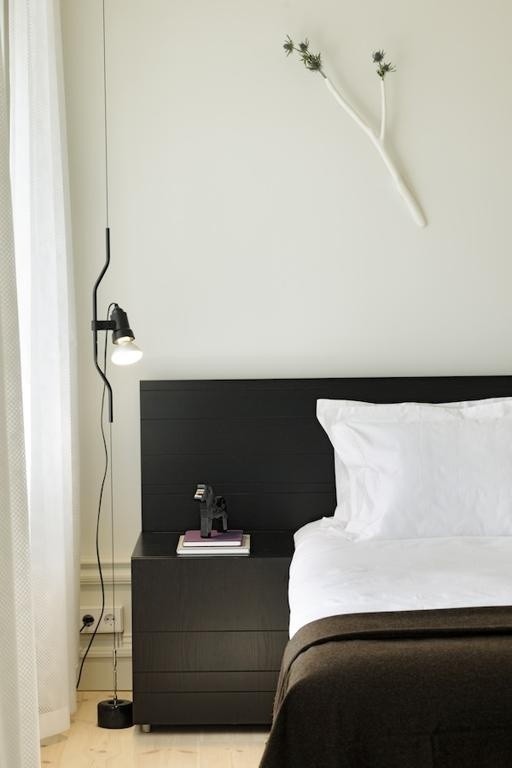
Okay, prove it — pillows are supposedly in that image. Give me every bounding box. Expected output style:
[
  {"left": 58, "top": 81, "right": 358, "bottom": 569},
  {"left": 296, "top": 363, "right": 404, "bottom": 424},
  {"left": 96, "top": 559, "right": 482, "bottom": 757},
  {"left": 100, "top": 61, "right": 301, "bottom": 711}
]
[{"left": 311, "top": 395, "right": 511, "bottom": 539}]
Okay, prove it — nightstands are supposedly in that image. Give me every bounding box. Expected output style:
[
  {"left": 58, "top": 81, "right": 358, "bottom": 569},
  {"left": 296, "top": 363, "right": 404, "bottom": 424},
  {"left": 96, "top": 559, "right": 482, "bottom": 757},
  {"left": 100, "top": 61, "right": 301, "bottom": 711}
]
[{"left": 130, "top": 530, "right": 294, "bottom": 733}]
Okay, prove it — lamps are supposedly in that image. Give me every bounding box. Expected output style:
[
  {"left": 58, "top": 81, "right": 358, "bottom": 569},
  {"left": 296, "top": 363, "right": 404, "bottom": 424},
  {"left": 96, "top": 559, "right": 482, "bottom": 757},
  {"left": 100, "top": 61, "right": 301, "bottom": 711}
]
[{"left": 87, "top": 228, "right": 141, "bottom": 420}]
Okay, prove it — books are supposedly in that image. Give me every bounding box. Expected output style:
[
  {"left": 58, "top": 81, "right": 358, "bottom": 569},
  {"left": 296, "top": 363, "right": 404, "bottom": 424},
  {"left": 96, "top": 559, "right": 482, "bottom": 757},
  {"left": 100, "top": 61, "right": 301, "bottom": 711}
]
[
  {"left": 182, "top": 528, "right": 243, "bottom": 547},
  {"left": 175, "top": 533, "right": 251, "bottom": 555}
]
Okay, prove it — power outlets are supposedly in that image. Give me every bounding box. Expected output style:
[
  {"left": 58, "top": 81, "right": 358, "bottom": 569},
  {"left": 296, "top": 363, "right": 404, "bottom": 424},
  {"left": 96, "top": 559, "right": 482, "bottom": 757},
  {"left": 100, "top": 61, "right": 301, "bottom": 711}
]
[{"left": 79, "top": 607, "right": 124, "bottom": 634}]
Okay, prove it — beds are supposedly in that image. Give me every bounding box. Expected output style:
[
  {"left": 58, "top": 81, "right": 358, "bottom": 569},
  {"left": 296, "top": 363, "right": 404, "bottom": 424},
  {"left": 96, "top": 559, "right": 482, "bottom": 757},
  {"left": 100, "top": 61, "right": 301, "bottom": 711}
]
[{"left": 139, "top": 378, "right": 511, "bottom": 768}]
